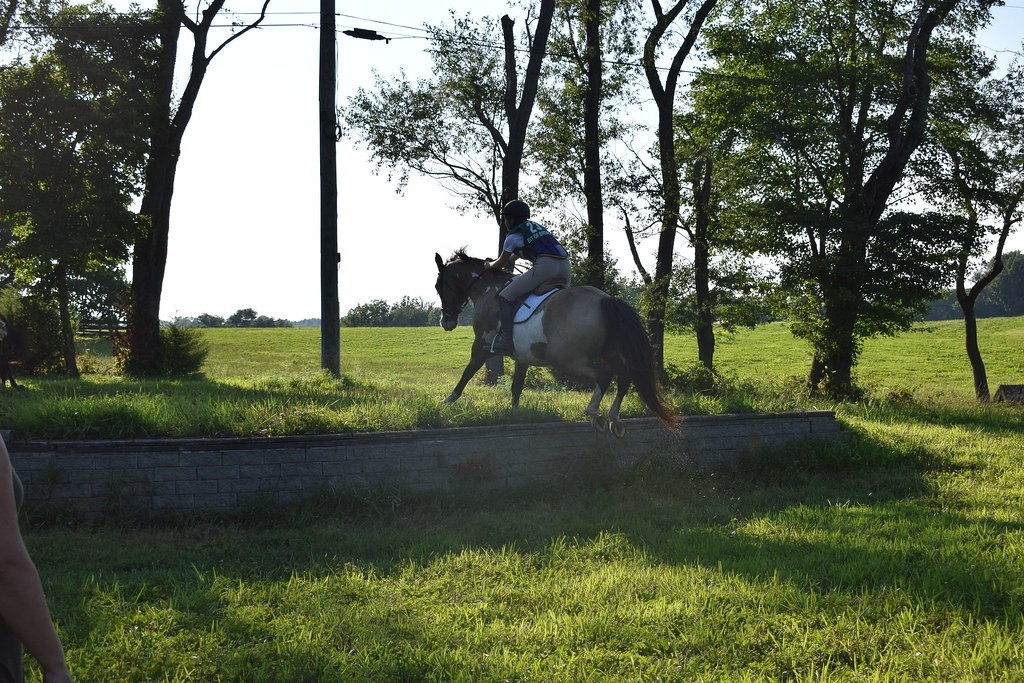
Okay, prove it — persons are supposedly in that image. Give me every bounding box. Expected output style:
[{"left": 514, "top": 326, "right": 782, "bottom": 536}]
[
  {"left": 482, "top": 200, "right": 570, "bottom": 357},
  {"left": 0, "top": 431, "right": 73, "bottom": 683}
]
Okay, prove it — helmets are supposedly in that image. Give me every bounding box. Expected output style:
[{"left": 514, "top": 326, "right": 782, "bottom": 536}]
[{"left": 500, "top": 200, "right": 531, "bottom": 219}]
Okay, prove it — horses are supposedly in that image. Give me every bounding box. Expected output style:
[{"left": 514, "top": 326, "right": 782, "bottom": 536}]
[{"left": 434, "top": 248, "right": 688, "bottom": 441}]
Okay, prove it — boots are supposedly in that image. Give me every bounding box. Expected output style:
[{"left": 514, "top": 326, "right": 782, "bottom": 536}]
[{"left": 482, "top": 295, "right": 517, "bottom": 351}]
